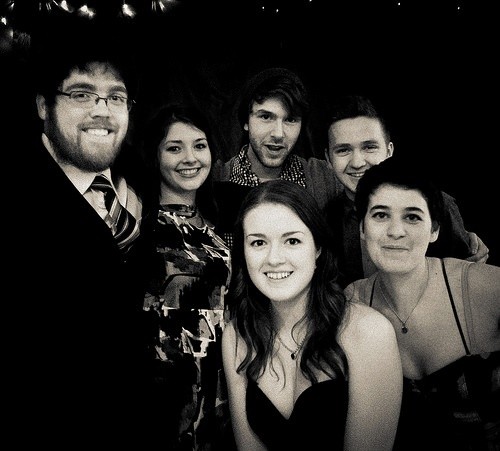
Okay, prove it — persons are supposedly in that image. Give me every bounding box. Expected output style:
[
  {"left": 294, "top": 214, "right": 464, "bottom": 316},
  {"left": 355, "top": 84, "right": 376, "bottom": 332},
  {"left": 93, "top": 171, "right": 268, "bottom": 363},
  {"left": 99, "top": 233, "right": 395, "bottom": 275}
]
[
  {"left": 221, "top": 180, "right": 404, "bottom": 451},
  {"left": 212, "top": 68, "right": 490, "bottom": 263},
  {"left": 342, "top": 157, "right": 500, "bottom": 451},
  {"left": 319, "top": 94, "right": 472, "bottom": 289},
  {"left": 0, "top": 47, "right": 236, "bottom": 451}
]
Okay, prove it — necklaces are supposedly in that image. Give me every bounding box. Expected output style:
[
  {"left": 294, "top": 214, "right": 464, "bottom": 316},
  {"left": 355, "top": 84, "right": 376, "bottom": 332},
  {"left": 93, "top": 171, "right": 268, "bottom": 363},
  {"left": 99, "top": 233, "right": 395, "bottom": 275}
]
[
  {"left": 375, "top": 257, "right": 431, "bottom": 333},
  {"left": 273, "top": 324, "right": 309, "bottom": 360}
]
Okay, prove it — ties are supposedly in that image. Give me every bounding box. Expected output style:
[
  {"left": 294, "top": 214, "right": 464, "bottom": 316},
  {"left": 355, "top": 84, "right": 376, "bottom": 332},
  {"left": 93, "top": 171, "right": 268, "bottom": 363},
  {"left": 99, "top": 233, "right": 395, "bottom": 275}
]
[{"left": 89, "top": 176, "right": 140, "bottom": 254}]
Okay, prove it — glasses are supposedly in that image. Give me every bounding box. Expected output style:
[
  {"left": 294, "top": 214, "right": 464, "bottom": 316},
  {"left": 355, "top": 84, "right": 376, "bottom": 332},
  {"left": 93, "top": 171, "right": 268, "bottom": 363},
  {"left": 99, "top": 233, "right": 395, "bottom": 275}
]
[{"left": 56, "top": 90, "right": 136, "bottom": 113}]
